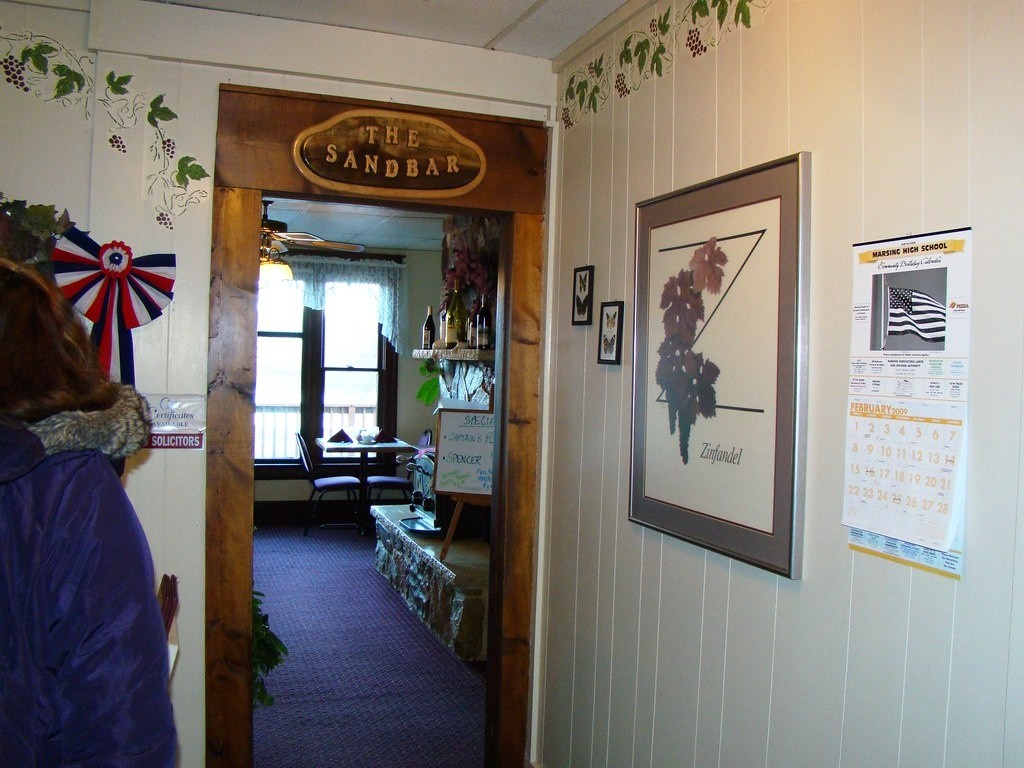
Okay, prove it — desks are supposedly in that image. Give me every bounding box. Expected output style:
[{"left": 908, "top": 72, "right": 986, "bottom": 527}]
[{"left": 314, "top": 437, "right": 416, "bottom": 528}]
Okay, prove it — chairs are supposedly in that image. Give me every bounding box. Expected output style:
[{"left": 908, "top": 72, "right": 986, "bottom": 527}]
[
  {"left": 294, "top": 432, "right": 368, "bottom": 538},
  {"left": 360, "top": 428, "right": 432, "bottom": 531}
]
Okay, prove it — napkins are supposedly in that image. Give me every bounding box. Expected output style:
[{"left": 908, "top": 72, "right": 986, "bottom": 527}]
[{"left": 328, "top": 429, "right": 352, "bottom": 442}]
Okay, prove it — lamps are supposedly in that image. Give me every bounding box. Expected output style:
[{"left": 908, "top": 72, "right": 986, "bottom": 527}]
[{"left": 259, "top": 227, "right": 295, "bottom": 284}]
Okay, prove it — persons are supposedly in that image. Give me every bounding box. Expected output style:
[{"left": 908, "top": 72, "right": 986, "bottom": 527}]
[{"left": 0, "top": 256, "right": 178, "bottom": 768}]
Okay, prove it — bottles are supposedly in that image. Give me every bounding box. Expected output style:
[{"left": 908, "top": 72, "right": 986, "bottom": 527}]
[
  {"left": 465, "top": 292, "right": 492, "bottom": 349},
  {"left": 439, "top": 279, "right": 462, "bottom": 349},
  {"left": 422, "top": 306, "right": 436, "bottom": 350}
]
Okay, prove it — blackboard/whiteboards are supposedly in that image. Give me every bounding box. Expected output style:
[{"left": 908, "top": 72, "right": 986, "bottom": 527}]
[{"left": 432, "top": 409, "right": 495, "bottom": 506}]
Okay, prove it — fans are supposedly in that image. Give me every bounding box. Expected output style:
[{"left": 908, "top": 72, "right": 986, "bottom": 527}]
[{"left": 256, "top": 199, "right": 368, "bottom": 255}]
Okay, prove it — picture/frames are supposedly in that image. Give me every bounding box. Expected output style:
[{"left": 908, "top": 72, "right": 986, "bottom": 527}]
[
  {"left": 628, "top": 152, "right": 812, "bottom": 580},
  {"left": 572, "top": 265, "right": 595, "bottom": 325},
  {"left": 595, "top": 300, "right": 625, "bottom": 365}
]
